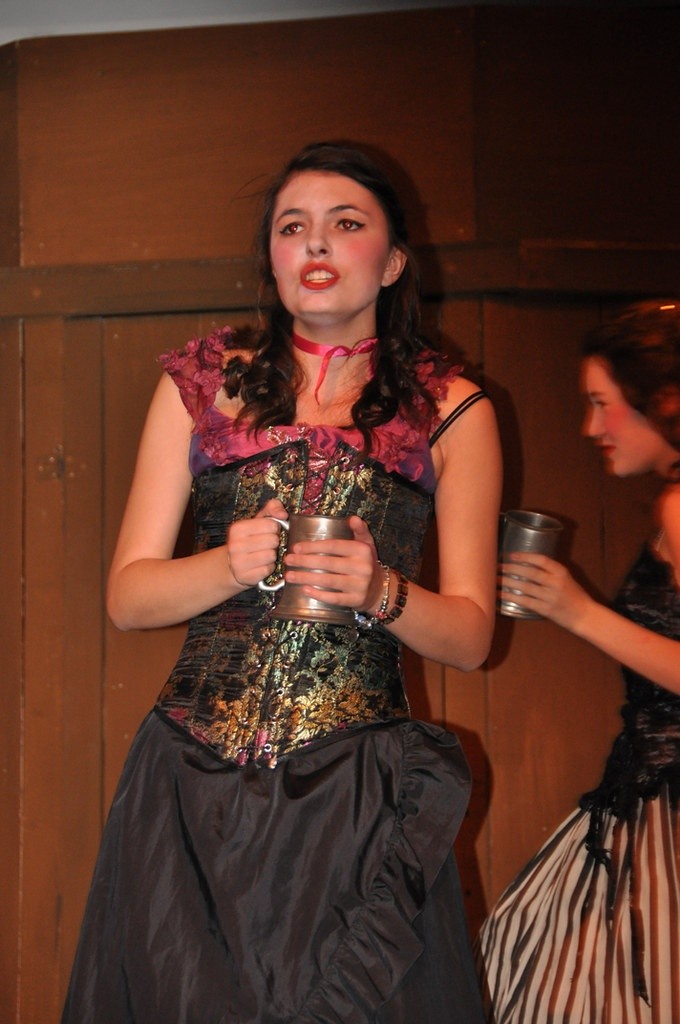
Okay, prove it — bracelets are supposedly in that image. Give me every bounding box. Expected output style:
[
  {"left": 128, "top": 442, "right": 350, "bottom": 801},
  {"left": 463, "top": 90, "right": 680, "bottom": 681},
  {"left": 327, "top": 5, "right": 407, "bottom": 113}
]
[
  {"left": 226, "top": 544, "right": 256, "bottom": 588},
  {"left": 353, "top": 561, "right": 408, "bottom": 629}
]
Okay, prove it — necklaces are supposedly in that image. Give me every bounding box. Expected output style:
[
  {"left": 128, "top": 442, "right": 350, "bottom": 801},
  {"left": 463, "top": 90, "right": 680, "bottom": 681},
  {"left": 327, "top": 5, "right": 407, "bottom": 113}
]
[{"left": 289, "top": 325, "right": 377, "bottom": 408}]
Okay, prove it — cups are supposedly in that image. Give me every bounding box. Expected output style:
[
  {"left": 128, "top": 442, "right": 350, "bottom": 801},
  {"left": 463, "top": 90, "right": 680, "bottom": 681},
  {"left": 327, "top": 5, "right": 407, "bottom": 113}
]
[
  {"left": 497, "top": 508, "right": 563, "bottom": 621},
  {"left": 256, "top": 513, "right": 359, "bottom": 626}
]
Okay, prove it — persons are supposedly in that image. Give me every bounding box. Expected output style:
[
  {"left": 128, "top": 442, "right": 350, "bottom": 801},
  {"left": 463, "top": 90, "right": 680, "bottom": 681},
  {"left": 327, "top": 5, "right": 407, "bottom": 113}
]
[
  {"left": 61, "top": 143, "right": 504, "bottom": 1023},
  {"left": 472, "top": 298, "right": 680, "bottom": 1023}
]
